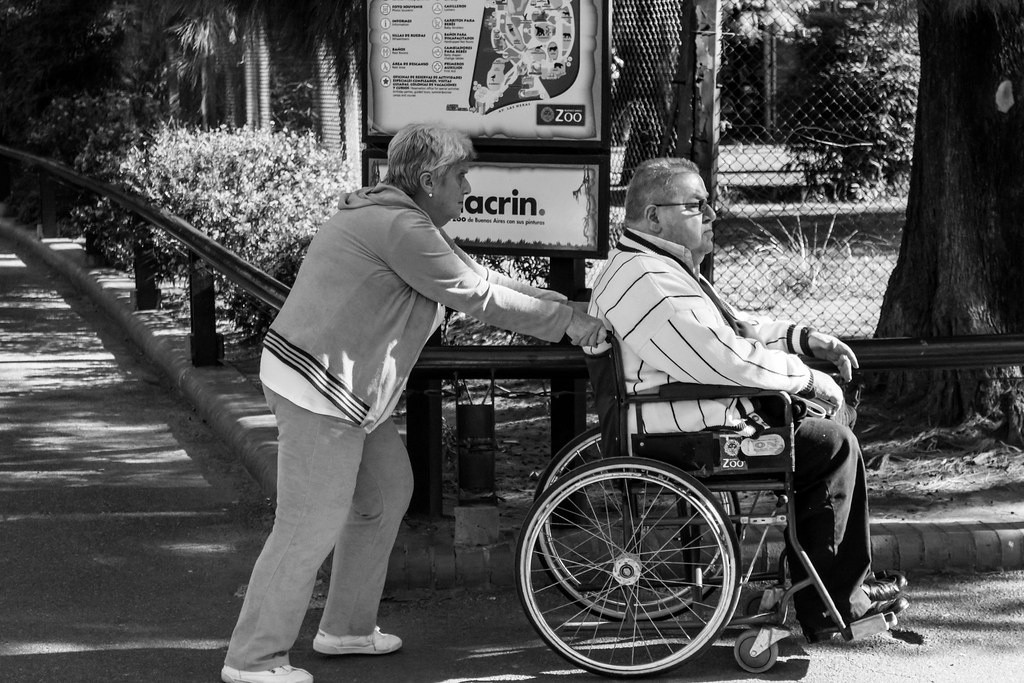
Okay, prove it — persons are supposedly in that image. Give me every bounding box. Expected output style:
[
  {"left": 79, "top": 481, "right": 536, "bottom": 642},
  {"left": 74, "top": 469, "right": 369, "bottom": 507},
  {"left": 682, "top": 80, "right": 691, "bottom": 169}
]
[
  {"left": 219, "top": 122, "right": 609, "bottom": 682},
  {"left": 581, "top": 156, "right": 911, "bottom": 647}
]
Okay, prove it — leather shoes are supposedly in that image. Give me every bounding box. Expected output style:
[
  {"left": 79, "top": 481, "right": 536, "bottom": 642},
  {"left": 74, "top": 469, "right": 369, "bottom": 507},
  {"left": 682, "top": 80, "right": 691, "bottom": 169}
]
[
  {"left": 806, "top": 597, "right": 909, "bottom": 644},
  {"left": 861, "top": 573, "right": 907, "bottom": 602}
]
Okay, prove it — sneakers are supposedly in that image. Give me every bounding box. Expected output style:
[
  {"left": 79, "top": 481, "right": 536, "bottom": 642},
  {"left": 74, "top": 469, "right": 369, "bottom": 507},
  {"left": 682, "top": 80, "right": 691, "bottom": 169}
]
[
  {"left": 313, "top": 625, "right": 403, "bottom": 654},
  {"left": 221, "top": 663, "right": 313, "bottom": 683}
]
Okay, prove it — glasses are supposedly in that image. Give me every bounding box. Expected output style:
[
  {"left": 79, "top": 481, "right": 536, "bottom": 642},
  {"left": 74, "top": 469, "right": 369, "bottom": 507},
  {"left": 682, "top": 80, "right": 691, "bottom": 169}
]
[{"left": 651, "top": 199, "right": 713, "bottom": 213}]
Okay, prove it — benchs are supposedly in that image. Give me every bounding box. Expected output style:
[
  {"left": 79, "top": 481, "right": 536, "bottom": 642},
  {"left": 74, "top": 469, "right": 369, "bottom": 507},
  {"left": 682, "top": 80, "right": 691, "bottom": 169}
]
[{"left": 608, "top": 143, "right": 907, "bottom": 225}]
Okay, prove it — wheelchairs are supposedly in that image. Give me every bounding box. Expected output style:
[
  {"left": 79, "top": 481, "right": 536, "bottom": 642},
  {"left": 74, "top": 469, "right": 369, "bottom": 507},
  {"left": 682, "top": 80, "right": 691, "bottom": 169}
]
[{"left": 510, "top": 332, "right": 923, "bottom": 678}]
